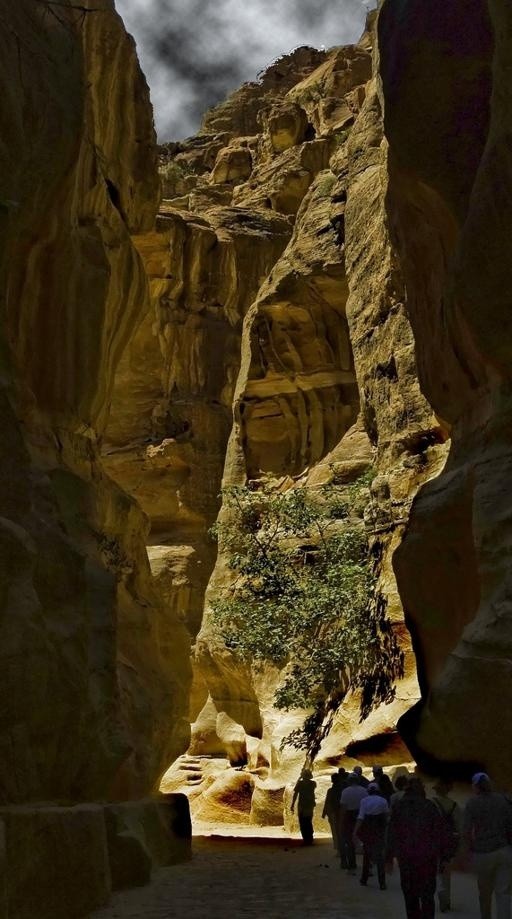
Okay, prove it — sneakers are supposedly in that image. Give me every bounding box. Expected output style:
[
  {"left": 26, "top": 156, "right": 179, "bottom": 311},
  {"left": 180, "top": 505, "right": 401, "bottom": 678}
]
[{"left": 343, "top": 868, "right": 394, "bottom": 892}]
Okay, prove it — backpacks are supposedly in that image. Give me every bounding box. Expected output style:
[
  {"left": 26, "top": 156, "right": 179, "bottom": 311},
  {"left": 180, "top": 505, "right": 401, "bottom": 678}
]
[{"left": 432, "top": 797, "right": 460, "bottom": 864}]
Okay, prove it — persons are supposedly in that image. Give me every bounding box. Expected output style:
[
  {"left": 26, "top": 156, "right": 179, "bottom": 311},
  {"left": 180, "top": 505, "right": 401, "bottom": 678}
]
[
  {"left": 462, "top": 772, "right": 511, "bottom": 918},
  {"left": 384, "top": 777, "right": 442, "bottom": 918},
  {"left": 321, "top": 765, "right": 406, "bottom": 891},
  {"left": 427, "top": 779, "right": 464, "bottom": 913},
  {"left": 289, "top": 769, "right": 317, "bottom": 845}
]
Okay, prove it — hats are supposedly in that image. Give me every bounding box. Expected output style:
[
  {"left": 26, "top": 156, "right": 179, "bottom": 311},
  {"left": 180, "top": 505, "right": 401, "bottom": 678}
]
[
  {"left": 471, "top": 772, "right": 490, "bottom": 786},
  {"left": 366, "top": 782, "right": 380, "bottom": 793},
  {"left": 432, "top": 777, "right": 453, "bottom": 790},
  {"left": 373, "top": 764, "right": 383, "bottom": 773},
  {"left": 353, "top": 766, "right": 363, "bottom": 775},
  {"left": 301, "top": 769, "right": 313, "bottom": 779},
  {"left": 344, "top": 773, "right": 361, "bottom": 786},
  {"left": 404, "top": 778, "right": 425, "bottom": 791}
]
